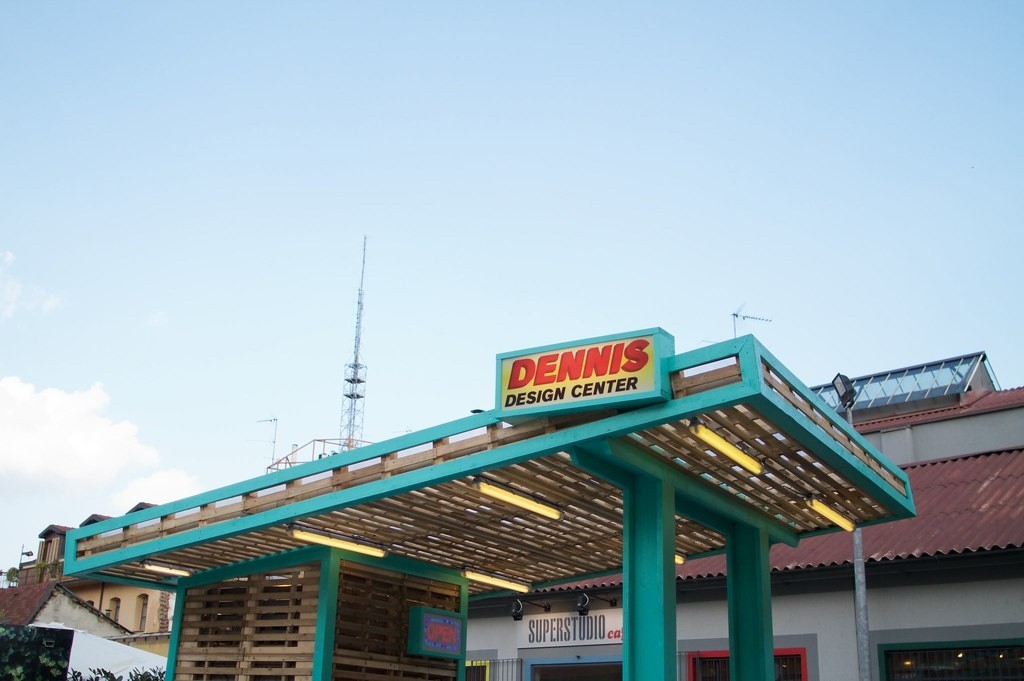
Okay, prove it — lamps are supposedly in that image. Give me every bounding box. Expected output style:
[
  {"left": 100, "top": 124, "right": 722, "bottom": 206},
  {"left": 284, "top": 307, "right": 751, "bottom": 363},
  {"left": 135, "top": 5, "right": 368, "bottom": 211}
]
[
  {"left": 510, "top": 598, "right": 551, "bottom": 621},
  {"left": 577, "top": 593, "right": 616, "bottom": 616}
]
